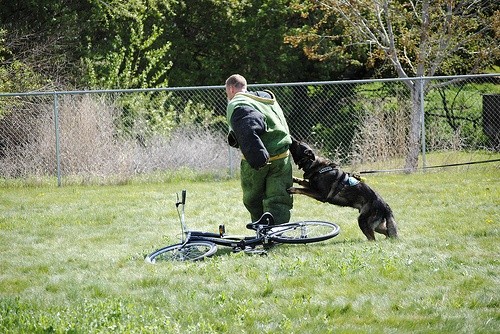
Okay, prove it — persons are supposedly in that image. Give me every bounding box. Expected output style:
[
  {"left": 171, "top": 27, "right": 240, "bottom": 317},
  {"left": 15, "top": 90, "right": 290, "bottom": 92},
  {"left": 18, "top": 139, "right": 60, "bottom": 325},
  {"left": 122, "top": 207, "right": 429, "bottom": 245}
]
[{"left": 224, "top": 73, "right": 294, "bottom": 225}]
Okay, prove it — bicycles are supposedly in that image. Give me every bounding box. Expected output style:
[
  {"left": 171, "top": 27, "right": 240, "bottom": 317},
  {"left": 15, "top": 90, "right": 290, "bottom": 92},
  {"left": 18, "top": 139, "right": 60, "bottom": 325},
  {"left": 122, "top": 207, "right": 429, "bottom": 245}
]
[{"left": 144, "top": 189, "right": 340, "bottom": 267}]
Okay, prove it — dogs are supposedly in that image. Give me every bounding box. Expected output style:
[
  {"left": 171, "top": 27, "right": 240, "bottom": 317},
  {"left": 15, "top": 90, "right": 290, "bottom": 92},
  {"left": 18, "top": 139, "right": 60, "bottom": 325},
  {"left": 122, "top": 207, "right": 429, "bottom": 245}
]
[{"left": 287, "top": 137, "right": 397, "bottom": 241}]
